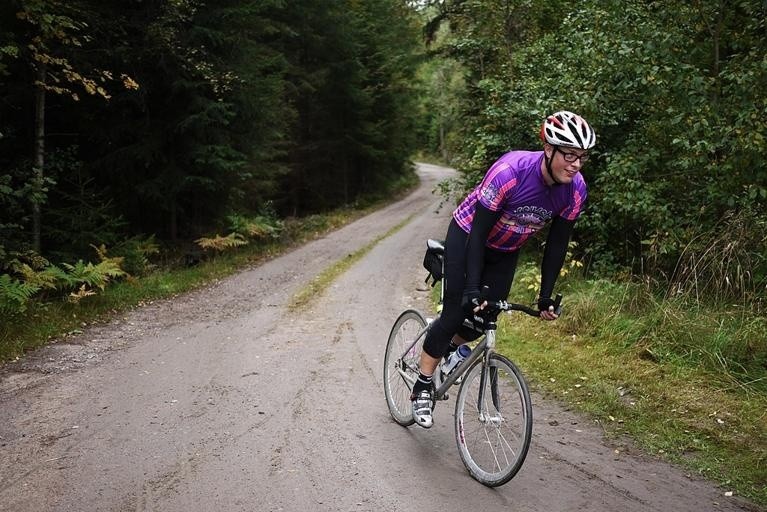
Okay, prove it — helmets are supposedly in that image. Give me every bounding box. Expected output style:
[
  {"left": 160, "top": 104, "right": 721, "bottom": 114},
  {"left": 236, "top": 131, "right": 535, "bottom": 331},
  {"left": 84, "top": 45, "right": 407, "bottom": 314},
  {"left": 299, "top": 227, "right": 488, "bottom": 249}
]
[{"left": 540, "top": 111, "right": 596, "bottom": 150}]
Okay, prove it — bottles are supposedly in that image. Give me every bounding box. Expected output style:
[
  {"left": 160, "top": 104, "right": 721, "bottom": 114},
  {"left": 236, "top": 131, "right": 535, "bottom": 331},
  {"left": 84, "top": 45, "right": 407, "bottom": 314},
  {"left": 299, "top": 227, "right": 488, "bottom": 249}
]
[{"left": 441, "top": 344, "right": 472, "bottom": 376}]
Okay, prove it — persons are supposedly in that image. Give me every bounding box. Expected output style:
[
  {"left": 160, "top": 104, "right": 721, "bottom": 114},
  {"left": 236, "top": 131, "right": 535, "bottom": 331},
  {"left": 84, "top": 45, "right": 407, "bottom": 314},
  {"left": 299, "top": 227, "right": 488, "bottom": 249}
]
[{"left": 411, "top": 111, "right": 599, "bottom": 429}]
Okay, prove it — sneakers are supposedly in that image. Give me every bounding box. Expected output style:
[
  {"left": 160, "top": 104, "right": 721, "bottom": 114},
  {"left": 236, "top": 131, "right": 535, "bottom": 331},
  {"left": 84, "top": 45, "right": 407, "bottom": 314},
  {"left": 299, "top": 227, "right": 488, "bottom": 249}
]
[
  {"left": 410, "top": 386, "right": 434, "bottom": 428},
  {"left": 442, "top": 354, "right": 463, "bottom": 384}
]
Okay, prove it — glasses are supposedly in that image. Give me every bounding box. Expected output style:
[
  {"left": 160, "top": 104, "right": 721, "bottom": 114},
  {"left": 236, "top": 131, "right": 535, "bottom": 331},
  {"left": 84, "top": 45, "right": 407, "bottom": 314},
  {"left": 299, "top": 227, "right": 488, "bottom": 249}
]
[{"left": 557, "top": 146, "right": 591, "bottom": 162}]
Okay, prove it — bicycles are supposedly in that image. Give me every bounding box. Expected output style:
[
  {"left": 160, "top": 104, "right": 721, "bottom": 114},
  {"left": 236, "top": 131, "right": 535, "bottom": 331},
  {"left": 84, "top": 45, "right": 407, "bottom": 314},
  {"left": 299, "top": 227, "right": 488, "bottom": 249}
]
[{"left": 382, "top": 239, "right": 562, "bottom": 487}]
